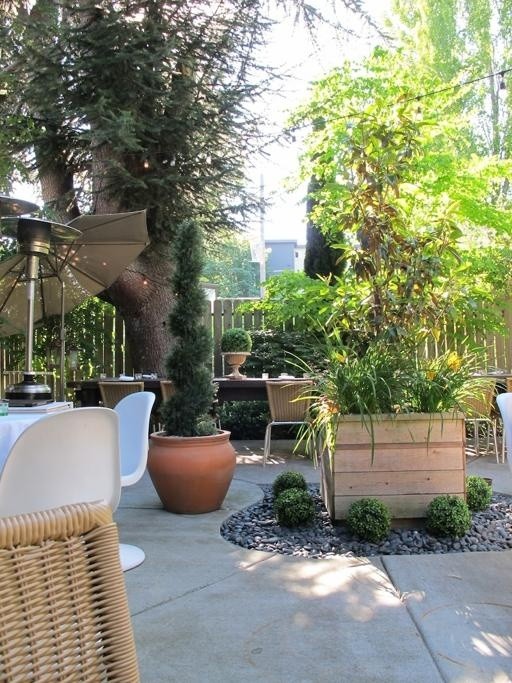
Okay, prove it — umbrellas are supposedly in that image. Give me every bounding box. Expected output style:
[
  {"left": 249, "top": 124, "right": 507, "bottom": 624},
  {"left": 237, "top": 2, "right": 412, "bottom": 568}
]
[{"left": 0, "top": 206, "right": 153, "bottom": 398}]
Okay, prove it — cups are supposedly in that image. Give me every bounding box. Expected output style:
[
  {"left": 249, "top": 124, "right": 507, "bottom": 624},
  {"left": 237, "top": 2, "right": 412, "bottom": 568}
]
[
  {"left": 261, "top": 371, "right": 311, "bottom": 378},
  {"left": 135, "top": 371, "right": 157, "bottom": 380},
  {"left": 100, "top": 372, "right": 106, "bottom": 379},
  {"left": 0, "top": 402, "right": 9, "bottom": 415}
]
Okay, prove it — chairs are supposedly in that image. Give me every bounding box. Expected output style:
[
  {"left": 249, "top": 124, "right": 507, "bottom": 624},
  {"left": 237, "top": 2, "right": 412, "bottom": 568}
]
[
  {"left": 108, "top": 390, "right": 159, "bottom": 571},
  {"left": 160, "top": 377, "right": 220, "bottom": 435},
  {"left": 263, "top": 374, "right": 314, "bottom": 461},
  {"left": 1, "top": 502, "right": 143, "bottom": 681},
  {"left": 1, "top": 407, "right": 124, "bottom": 525},
  {"left": 457, "top": 373, "right": 511, "bottom": 467},
  {"left": 103, "top": 380, "right": 143, "bottom": 405}
]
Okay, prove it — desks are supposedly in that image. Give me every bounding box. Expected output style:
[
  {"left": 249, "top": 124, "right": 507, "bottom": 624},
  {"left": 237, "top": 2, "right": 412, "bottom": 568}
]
[
  {"left": 1, "top": 403, "right": 74, "bottom": 468},
  {"left": 65, "top": 371, "right": 512, "bottom": 462}
]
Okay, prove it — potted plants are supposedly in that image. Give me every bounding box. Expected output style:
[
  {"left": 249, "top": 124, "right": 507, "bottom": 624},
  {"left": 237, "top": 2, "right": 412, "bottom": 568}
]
[
  {"left": 237, "top": 26, "right": 511, "bottom": 532},
  {"left": 147, "top": 222, "right": 237, "bottom": 517},
  {"left": 220, "top": 329, "right": 252, "bottom": 378}
]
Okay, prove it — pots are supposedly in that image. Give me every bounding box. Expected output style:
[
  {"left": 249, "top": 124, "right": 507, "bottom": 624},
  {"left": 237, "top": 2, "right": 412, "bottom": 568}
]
[{"left": 4, "top": 370, "right": 53, "bottom": 407}]
